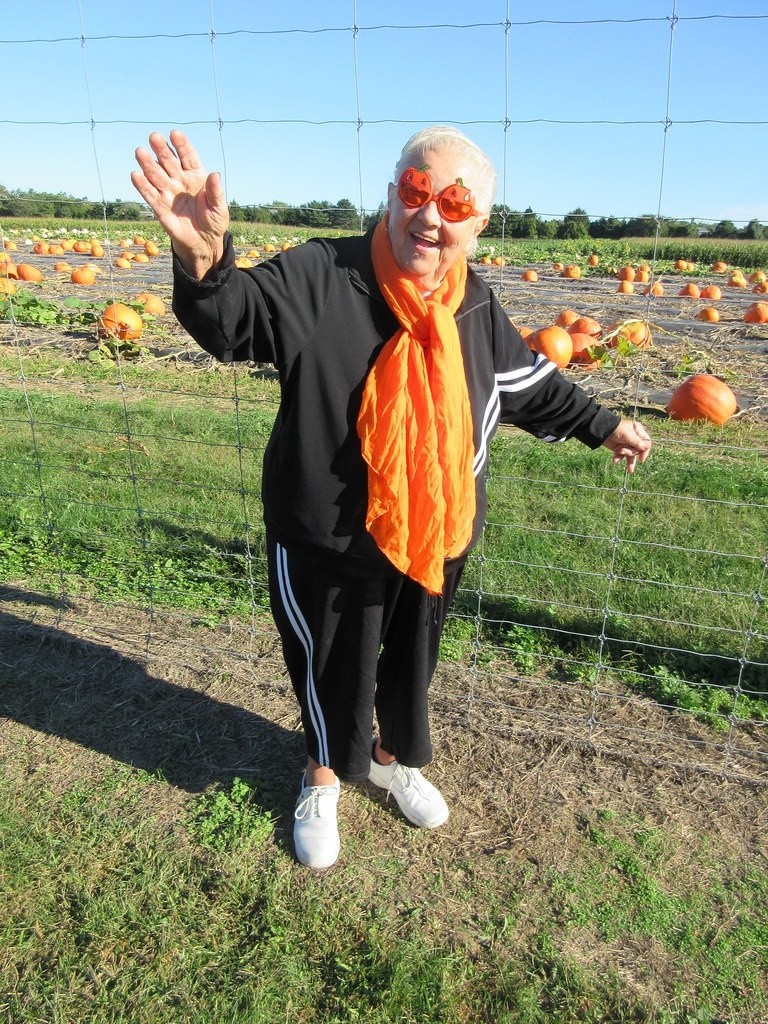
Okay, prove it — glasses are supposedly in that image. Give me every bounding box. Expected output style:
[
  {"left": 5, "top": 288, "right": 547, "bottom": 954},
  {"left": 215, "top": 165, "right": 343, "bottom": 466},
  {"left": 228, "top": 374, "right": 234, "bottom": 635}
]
[{"left": 397, "top": 165, "right": 484, "bottom": 222}]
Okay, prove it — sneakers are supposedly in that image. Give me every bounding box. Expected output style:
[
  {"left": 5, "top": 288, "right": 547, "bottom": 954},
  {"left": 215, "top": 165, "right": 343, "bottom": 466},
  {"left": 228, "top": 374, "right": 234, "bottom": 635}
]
[
  {"left": 293, "top": 769, "right": 340, "bottom": 868},
  {"left": 367, "top": 737, "right": 449, "bottom": 828}
]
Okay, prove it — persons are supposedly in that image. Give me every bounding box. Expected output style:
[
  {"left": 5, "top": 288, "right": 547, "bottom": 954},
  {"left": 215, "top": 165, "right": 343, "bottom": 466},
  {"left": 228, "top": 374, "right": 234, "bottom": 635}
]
[{"left": 129, "top": 125, "right": 652, "bottom": 872}]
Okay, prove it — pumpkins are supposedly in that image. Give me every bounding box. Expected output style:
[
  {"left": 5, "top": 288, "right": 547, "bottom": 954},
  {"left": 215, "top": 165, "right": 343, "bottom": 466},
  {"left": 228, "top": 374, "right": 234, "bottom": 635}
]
[
  {"left": 480, "top": 252, "right": 768, "bottom": 428},
  {"left": 0, "top": 227, "right": 166, "bottom": 341},
  {"left": 234, "top": 235, "right": 306, "bottom": 268}
]
